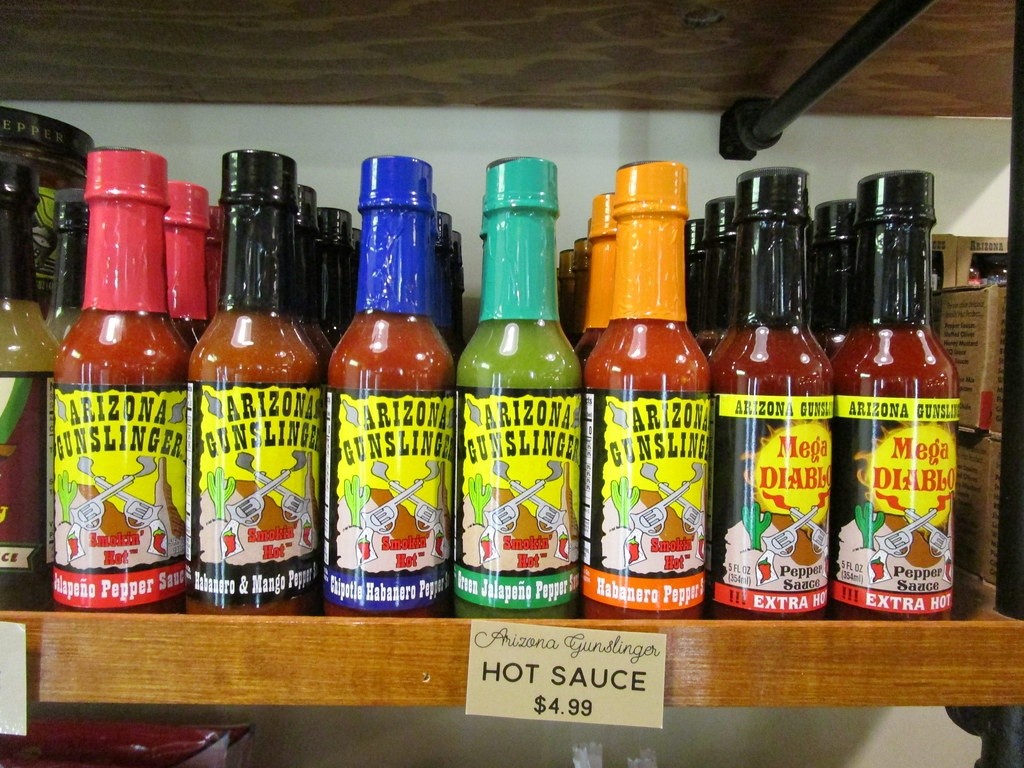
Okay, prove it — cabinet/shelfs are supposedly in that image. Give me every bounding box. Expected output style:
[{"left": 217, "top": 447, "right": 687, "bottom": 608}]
[{"left": 0, "top": 0, "right": 1024, "bottom": 711}]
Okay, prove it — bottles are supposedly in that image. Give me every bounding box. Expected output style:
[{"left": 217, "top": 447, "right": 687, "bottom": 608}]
[
  {"left": 969, "top": 269, "right": 1007, "bottom": 287},
  {"left": 0, "top": 149, "right": 959, "bottom": 620}
]
